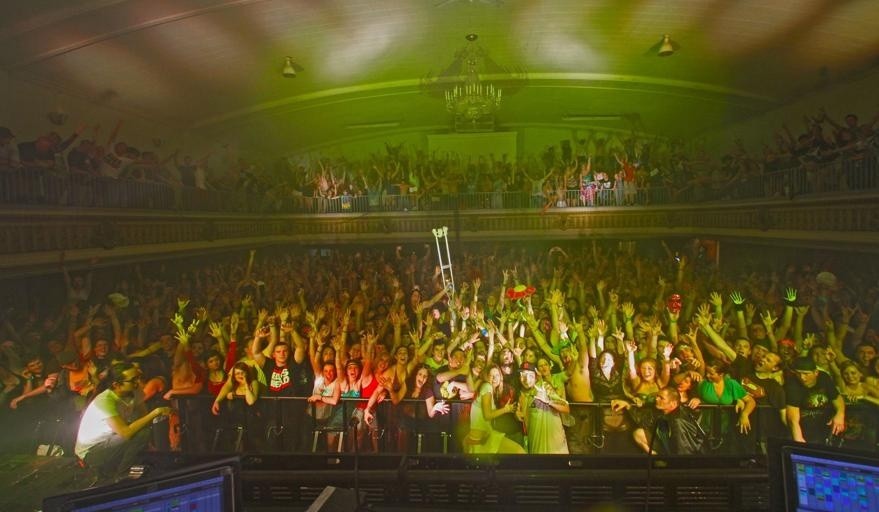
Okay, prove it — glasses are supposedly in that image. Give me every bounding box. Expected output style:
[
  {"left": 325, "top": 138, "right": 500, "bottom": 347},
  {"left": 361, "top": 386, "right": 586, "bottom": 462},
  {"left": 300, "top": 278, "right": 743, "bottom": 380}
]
[{"left": 118, "top": 377, "right": 139, "bottom": 383}]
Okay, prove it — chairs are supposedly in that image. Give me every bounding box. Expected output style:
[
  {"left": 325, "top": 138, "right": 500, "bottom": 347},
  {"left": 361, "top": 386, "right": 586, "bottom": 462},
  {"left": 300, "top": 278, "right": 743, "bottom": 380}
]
[{"left": 172, "top": 393, "right": 875, "bottom": 469}]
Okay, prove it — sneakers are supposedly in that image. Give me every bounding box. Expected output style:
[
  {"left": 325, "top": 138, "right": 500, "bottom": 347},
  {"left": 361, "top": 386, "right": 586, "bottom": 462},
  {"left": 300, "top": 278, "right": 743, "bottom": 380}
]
[{"left": 94, "top": 474, "right": 129, "bottom": 488}]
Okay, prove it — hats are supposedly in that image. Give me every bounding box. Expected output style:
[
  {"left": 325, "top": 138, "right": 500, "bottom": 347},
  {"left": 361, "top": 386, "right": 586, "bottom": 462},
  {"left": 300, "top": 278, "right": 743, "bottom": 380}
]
[
  {"left": 516, "top": 361, "right": 537, "bottom": 372},
  {"left": 754, "top": 338, "right": 771, "bottom": 351},
  {"left": 792, "top": 357, "right": 817, "bottom": 371}
]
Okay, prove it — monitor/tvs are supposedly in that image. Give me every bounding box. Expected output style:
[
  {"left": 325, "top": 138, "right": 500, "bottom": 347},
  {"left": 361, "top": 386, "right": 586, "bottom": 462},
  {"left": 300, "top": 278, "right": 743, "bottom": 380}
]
[
  {"left": 42, "top": 454, "right": 242, "bottom": 512},
  {"left": 773, "top": 439, "right": 879, "bottom": 512}
]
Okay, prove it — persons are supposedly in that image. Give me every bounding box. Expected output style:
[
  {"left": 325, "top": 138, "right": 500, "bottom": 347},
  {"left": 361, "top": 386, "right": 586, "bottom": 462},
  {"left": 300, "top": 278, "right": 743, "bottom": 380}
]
[{"left": 0, "top": 105, "right": 877, "bottom": 486}]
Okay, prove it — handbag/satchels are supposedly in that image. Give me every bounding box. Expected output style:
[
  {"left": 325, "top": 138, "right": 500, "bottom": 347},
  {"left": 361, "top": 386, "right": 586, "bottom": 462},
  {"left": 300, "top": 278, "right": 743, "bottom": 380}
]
[{"left": 464, "top": 430, "right": 490, "bottom": 445}]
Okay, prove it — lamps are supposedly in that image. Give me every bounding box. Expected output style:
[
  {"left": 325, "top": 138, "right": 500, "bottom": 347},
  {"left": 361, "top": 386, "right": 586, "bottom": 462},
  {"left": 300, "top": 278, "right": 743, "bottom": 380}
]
[
  {"left": 657, "top": 33, "right": 675, "bottom": 55},
  {"left": 417, "top": 34, "right": 529, "bottom": 122},
  {"left": 281, "top": 56, "right": 296, "bottom": 79}
]
